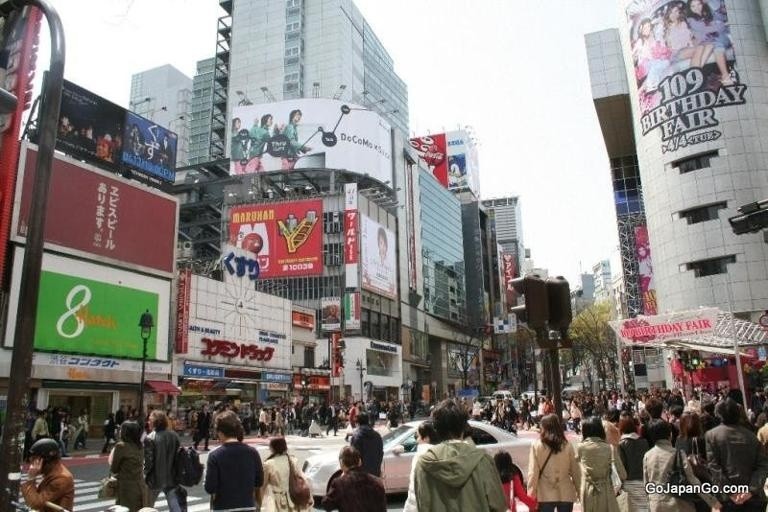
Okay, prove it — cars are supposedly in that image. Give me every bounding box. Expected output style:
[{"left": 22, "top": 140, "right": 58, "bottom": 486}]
[
  {"left": 298, "top": 417, "right": 534, "bottom": 500},
  {"left": 428, "top": 382, "right": 588, "bottom": 420}
]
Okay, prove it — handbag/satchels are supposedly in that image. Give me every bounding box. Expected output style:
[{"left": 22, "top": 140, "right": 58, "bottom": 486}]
[
  {"left": 661, "top": 465, "right": 697, "bottom": 500},
  {"left": 609, "top": 444, "right": 623, "bottom": 495},
  {"left": 287, "top": 451, "right": 311, "bottom": 505},
  {"left": 685, "top": 437, "right": 706, "bottom": 472},
  {"left": 98, "top": 474, "right": 119, "bottom": 500}
]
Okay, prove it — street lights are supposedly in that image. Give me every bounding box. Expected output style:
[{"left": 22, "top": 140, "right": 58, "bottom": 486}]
[
  {"left": 356, "top": 358, "right": 368, "bottom": 403},
  {"left": 729, "top": 197, "right": 768, "bottom": 237},
  {"left": 137, "top": 307, "right": 154, "bottom": 419}
]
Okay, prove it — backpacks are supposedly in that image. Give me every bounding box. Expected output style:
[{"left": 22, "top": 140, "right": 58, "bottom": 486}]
[{"left": 171, "top": 431, "right": 204, "bottom": 487}]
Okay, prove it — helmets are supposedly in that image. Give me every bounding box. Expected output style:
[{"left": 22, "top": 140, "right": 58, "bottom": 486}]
[{"left": 28, "top": 438, "right": 61, "bottom": 460}]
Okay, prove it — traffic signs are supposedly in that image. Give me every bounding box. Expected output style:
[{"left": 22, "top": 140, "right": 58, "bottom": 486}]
[{"left": 759, "top": 314, "right": 768, "bottom": 329}]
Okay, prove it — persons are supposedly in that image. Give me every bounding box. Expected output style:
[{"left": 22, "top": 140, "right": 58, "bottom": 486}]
[
  {"left": 449, "top": 156, "right": 462, "bottom": 177},
  {"left": 232, "top": 117, "right": 247, "bottom": 175},
  {"left": 1, "top": 386, "right": 765, "bottom": 511},
  {"left": 686, "top": 0, "right": 731, "bottom": 78},
  {"left": 364, "top": 228, "right": 395, "bottom": 294},
  {"left": 60, "top": 114, "right": 122, "bottom": 163},
  {"left": 637, "top": 245, "right": 652, "bottom": 278},
  {"left": 632, "top": 17, "right": 669, "bottom": 92},
  {"left": 665, "top": 6, "right": 713, "bottom": 69},
  {"left": 245, "top": 113, "right": 284, "bottom": 173},
  {"left": 128, "top": 123, "right": 146, "bottom": 156},
  {"left": 326, "top": 304, "right": 339, "bottom": 323},
  {"left": 281, "top": 109, "right": 312, "bottom": 170},
  {"left": 157, "top": 134, "right": 172, "bottom": 166}
]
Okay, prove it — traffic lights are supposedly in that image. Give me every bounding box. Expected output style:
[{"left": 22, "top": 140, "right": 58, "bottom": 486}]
[
  {"left": 543, "top": 272, "right": 575, "bottom": 331},
  {"left": 507, "top": 273, "right": 549, "bottom": 336},
  {"left": 677, "top": 349, "right": 688, "bottom": 367},
  {"left": 690, "top": 349, "right": 701, "bottom": 367},
  {"left": 0, "top": 85, "right": 20, "bottom": 117}
]
